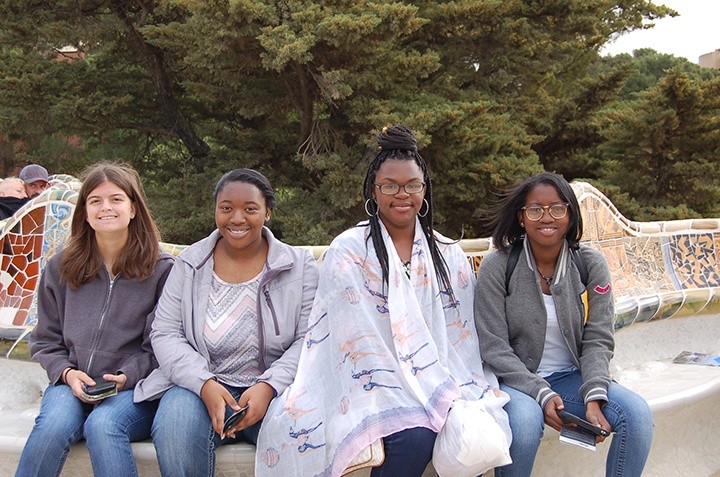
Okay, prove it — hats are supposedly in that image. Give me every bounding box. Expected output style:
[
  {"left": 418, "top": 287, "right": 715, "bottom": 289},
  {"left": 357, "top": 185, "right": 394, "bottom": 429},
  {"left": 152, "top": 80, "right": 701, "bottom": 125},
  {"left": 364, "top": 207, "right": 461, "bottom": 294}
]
[{"left": 19, "top": 164, "right": 48, "bottom": 183}]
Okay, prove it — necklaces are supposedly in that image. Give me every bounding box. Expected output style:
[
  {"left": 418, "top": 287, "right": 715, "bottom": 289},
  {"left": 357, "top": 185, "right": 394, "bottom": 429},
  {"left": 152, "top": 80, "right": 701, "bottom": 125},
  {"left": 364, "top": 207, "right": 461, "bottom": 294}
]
[{"left": 536, "top": 261, "right": 555, "bottom": 287}]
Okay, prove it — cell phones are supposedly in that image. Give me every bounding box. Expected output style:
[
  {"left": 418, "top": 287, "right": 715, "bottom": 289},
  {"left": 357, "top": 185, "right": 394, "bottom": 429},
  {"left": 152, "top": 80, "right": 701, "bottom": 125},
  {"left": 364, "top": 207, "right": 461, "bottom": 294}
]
[
  {"left": 556, "top": 409, "right": 611, "bottom": 438},
  {"left": 84, "top": 376, "right": 118, "bottom": 396}
]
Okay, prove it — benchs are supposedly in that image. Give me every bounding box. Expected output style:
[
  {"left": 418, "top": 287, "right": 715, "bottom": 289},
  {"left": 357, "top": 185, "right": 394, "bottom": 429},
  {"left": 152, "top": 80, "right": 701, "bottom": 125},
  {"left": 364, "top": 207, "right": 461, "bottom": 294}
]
[{"left": 0, "top": 176, "right": 720, "bottom": 477}]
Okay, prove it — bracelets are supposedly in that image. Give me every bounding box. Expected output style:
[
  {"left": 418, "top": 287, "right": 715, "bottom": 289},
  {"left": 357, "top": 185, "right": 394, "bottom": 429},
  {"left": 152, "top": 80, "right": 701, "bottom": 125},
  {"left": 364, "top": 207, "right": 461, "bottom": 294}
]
[{"left": 65, "top": 368, "right": 76, "bottom": 388}]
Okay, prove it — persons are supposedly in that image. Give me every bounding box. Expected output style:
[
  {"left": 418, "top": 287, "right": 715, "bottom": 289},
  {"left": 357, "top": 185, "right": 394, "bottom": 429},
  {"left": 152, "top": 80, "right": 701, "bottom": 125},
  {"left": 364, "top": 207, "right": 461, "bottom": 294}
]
[
  {"left": 0, "top": 164, "right": 50, "bottom": 221},
  {"left": 133, "top": 168, "right": 320, "bottom": 477},
  {"left": 254, "top": 125, "right": 513, "bottom": 477},
  {"left": 473, "top": 172, "right": 652, "bottom": 477},
  {"left": 14, "top": 164, "right": 176, "bottom": 477}
]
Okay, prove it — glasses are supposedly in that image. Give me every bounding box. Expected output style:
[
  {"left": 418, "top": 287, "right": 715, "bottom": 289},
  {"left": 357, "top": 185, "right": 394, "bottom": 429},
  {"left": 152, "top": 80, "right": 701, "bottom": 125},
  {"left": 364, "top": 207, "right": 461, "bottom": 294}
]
[
  {"left": 373, "top": 181, "right": 428, "bottom": 195},
  {"left": 522, "top": 202, "right": 570, "bottom": 221}
]
